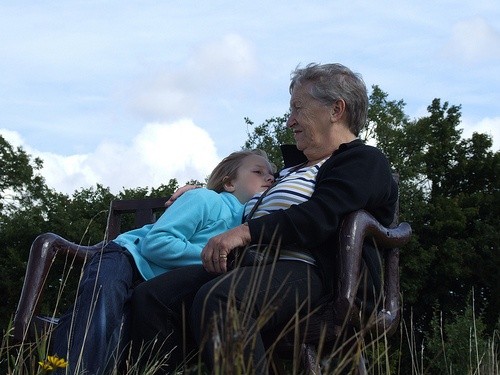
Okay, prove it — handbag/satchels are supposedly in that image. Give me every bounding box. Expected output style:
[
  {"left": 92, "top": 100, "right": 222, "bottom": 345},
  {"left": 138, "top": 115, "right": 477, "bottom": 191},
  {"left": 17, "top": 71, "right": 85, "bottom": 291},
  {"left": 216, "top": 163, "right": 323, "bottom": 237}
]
[{"left": 224, "top": 241, "right": 276, "bottom": 267}]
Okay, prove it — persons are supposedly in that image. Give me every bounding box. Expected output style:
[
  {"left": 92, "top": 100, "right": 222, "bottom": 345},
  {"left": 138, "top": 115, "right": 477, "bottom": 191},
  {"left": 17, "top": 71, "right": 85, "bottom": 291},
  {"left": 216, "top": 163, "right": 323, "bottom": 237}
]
[
  {"left": 126, "top": 62, "right": 399, "bottom": 375},
  {"left": 47, "top": 150, "right": 281, "bottom": 375}
]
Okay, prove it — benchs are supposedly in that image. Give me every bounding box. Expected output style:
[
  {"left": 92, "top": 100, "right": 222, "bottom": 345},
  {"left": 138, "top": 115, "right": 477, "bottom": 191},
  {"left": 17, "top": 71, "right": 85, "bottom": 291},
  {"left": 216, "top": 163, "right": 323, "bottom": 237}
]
[{"left": 14, "top": 173, "right": 412, "bottom": 375}]
